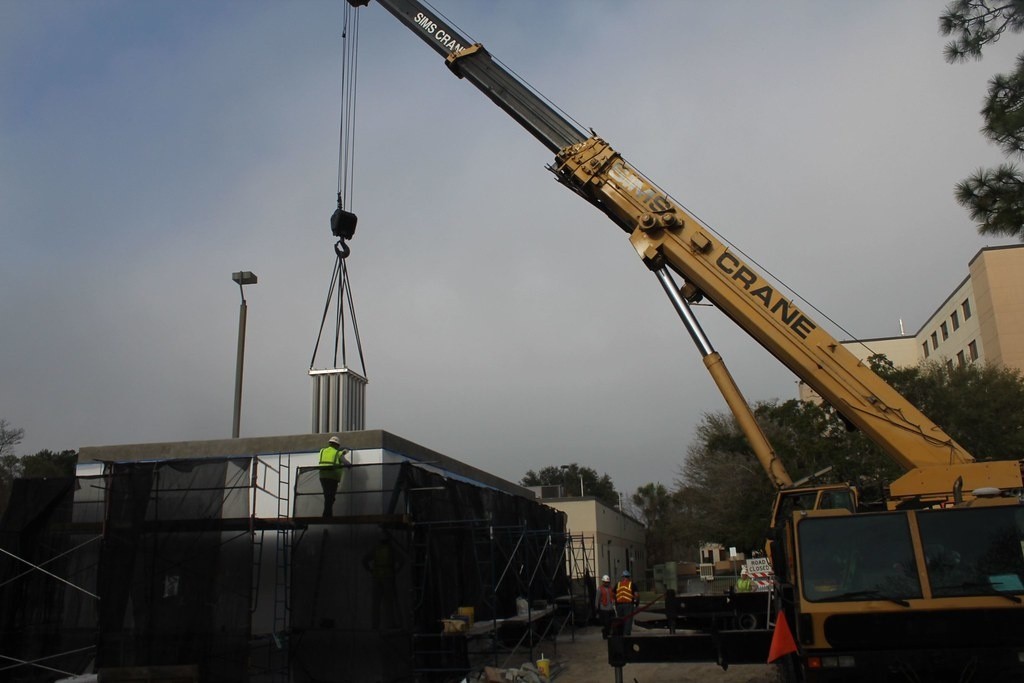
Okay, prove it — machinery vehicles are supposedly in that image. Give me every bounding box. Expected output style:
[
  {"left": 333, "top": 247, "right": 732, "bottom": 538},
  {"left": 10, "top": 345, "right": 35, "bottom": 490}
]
[{"left": 328, "top": 0, "right": 1024, "bottom": 683}]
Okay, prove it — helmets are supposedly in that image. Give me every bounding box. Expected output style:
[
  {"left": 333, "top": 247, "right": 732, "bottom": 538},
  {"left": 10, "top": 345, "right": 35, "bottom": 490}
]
[
  {"left": 328, "top": 436, "right": 340, "bottom": 445},
  {"left": 622, "top": 570, "right": 631, "bottom": 577},
  {"left": 740, "top": 570, "right": 748, "bottom": 575},
  {"left": 602, "top": 575, "right": 610, "bottom": 582}
]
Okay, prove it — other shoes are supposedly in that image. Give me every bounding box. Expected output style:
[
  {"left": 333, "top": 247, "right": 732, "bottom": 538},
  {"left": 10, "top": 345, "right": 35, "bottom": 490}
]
[{"left": 601, "top": 629, "right": 608, "bottom": 639}]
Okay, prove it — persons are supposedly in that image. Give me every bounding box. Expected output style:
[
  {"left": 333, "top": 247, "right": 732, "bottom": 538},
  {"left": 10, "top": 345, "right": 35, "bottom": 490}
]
[
  {"left": 596, "top": 574, "right": 615, "bottom": 639},
  {"left": 735, "top": 567, "right": 758, "bottom": 593},
  {"left": 317, "top": 436, "right": 351, "bottom": 516},
  {"left": 612, "top": 570, "right": 640, "bottom": 636}
]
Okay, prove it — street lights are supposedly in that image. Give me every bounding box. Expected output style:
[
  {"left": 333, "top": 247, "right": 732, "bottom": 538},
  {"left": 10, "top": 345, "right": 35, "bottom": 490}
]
[{"left": 233, "top": 271, "right": 258, "bottom": 437}]
